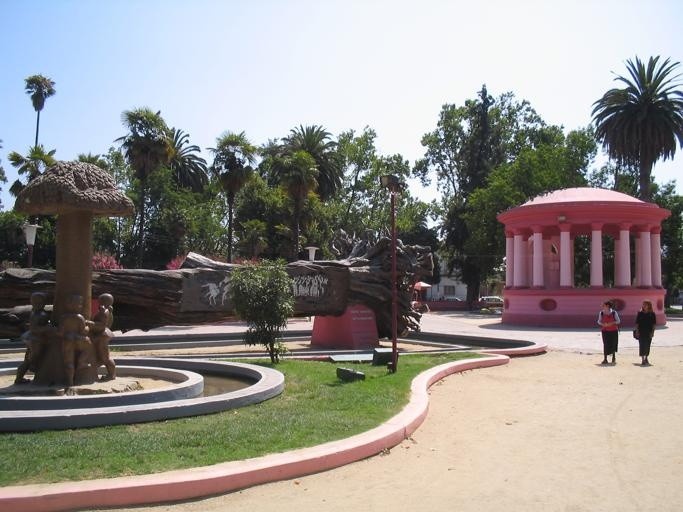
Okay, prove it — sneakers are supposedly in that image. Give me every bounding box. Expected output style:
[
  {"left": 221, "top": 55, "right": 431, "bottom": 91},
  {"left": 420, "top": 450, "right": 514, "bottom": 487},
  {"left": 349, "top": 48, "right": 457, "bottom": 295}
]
[
  {"left": 612, "top": 357, "right": 616, "bottom": 362},
  {"left": 642, "top": 359, "right": 648, "bottom": 365},
  {"left": 602, "top": 359, "right": 608, "bottom": 364}
]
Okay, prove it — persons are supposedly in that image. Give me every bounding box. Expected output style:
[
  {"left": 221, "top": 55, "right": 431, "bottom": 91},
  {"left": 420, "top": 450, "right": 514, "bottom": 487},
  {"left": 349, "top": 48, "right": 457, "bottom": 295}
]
[
  {"left": 635, "top": 300, "right": 656, "bottom": 365},
  {"left": 14, "top": 291, "right": 117, "bottom": 387},
  {"left": 596, "top": 300, "right": 621, "bottom": 364}
]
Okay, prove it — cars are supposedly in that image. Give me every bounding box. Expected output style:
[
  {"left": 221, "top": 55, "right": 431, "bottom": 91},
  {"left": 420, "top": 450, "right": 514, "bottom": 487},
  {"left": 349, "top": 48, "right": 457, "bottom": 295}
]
[{"left": 444, "top": 297, "right": 461, "bottom": 302}]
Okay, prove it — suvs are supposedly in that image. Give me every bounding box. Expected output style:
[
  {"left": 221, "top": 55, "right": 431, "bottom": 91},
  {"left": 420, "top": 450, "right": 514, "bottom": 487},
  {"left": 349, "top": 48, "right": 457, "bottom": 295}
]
[{"left": 478, "top": 296, "right": 503, "bottom": 306}]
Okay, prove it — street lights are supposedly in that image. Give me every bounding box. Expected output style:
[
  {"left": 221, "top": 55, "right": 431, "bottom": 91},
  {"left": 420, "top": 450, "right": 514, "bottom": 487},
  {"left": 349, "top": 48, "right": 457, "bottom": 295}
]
[
  {"left": 379, "top": 173, "right": 405, "bottom": 370},
  {"left": 20, "top": 224, "right": 43, "bottom": 267},
  {"left": 303, "top": 245, "right": 319, "bottom": 262}
]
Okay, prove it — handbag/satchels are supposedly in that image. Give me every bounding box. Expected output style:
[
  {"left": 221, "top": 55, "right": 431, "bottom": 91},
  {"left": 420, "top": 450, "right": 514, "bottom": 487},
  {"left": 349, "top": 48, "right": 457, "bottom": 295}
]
[{"left": 633, "top": 327, "right": 639, "bottom": 340}]
